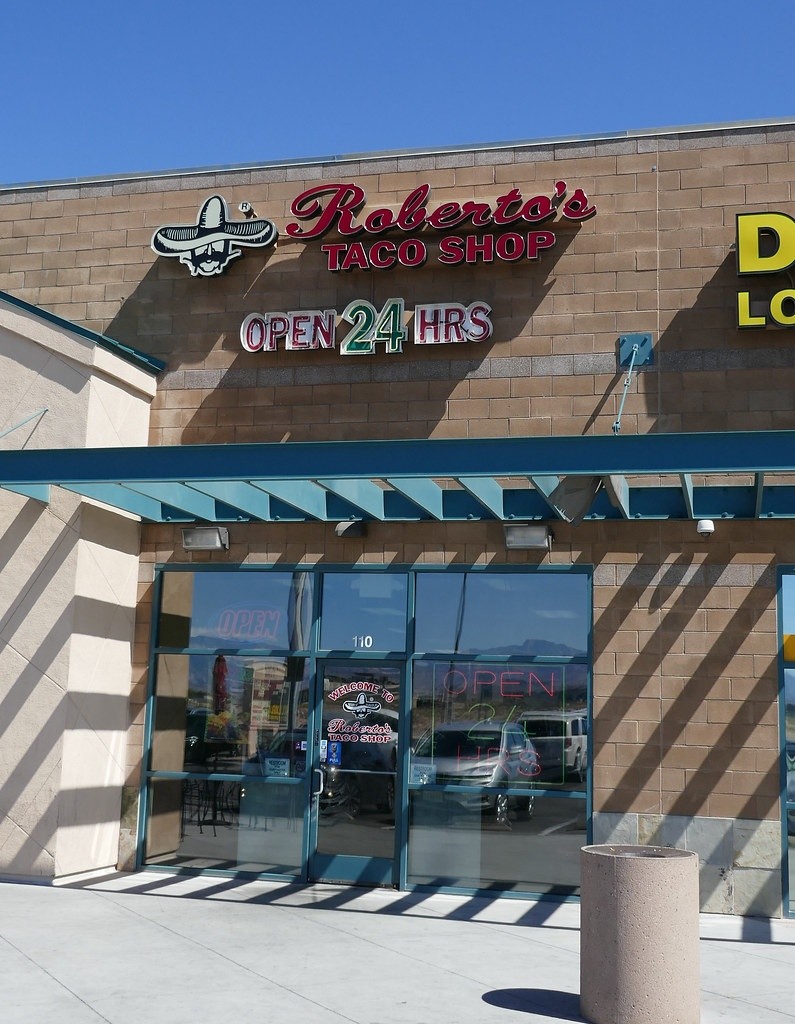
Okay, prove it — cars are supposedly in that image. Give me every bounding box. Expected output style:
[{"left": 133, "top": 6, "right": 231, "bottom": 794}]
[{"left": 269, "top": 707, "right": 399, "bottom": 812}]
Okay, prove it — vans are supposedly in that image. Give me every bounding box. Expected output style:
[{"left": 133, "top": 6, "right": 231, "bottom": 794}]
[{"left": 516, "top": 710, "right": 588, "bottom": 783}]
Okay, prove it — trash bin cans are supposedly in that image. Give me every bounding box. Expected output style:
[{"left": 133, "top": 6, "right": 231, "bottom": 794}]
[{"left": 579, "top": 844, "right": 700, "bottom": 1024}]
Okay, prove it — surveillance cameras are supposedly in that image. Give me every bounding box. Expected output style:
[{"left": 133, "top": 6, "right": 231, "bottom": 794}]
[{"left": 697, "top": 520, "right": 715, "bottom": 538}]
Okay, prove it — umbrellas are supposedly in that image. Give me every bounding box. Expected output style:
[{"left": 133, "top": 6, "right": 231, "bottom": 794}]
[
  {"left": 212, "top": 653, "right": 228, "bottom": 716},
  {"left": 287, "top": 571, "right": 312, "bottom": 651}
]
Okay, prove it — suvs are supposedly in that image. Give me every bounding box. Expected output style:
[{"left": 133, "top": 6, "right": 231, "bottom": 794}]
[
  {"left": 408, "top": 721, "right": 537, "bottom": 824},
  {"left": 187, "top": 708, "right": 243, "bottom": 759}
]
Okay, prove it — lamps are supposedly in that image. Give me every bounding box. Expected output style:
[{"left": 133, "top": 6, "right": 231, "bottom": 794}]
[
  {"left": 505, "top": 523, "right": 554, "bottom": 550},
  {"left": 179, "top": 526, "right": 230, "bottom": 551},
  {"left": 334, "top": 521, "right": 366, "bottom": 538}
]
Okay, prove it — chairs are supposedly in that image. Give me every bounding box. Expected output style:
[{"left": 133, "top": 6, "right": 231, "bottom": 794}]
[{"left": 180, "top": 736, "right": 300, "bottom": 836}]
